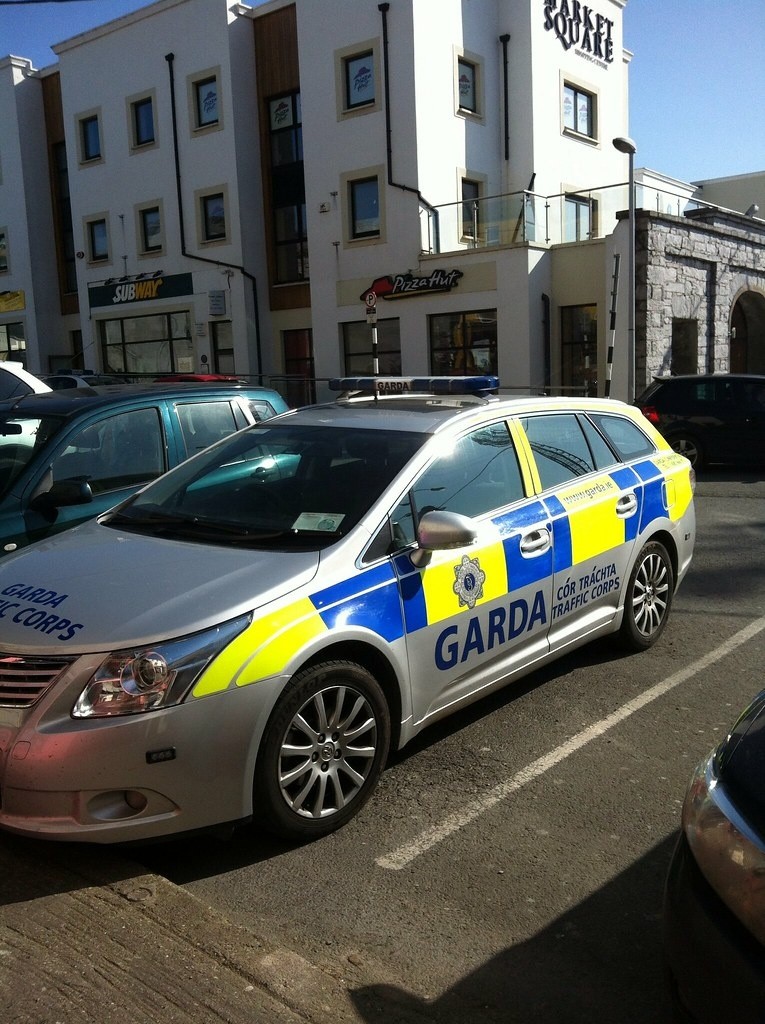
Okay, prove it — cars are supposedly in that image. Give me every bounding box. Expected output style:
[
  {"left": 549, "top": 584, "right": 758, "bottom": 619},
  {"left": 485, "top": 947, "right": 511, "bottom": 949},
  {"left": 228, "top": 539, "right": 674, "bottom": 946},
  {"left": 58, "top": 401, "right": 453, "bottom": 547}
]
[
  {"left": 1, "top": 378, "right": 697, "bottom": 846},
  {"left": 634, "top": 373, "right": 764, "bottom": 472},
  {"left": 0, "top": 360, "right": 292, "bottom": 558}
]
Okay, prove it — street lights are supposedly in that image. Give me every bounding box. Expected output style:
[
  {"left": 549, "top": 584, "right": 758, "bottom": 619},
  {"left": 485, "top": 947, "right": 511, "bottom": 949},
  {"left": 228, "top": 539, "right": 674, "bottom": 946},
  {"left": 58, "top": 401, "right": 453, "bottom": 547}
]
[{"left": 612, "top": 136, "right": 636, "bottom": 405}]
